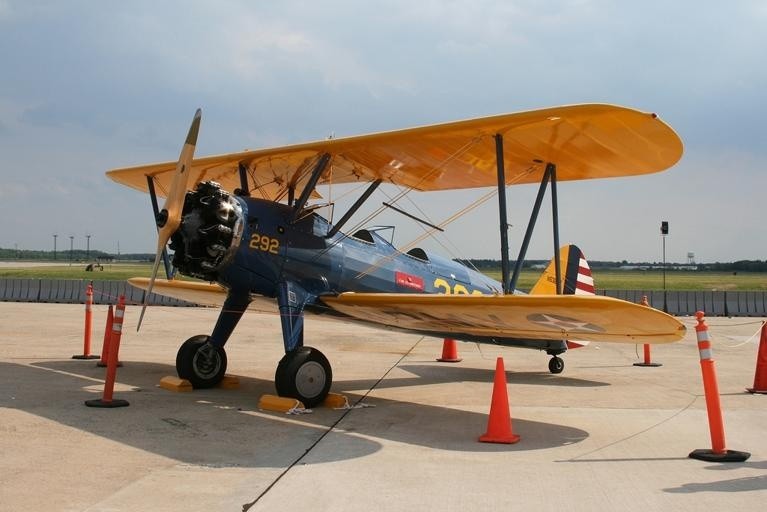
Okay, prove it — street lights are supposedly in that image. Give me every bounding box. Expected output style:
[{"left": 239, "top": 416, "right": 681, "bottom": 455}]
[{"left": 659, "top": 222, "right": 666, "bottom": 291}]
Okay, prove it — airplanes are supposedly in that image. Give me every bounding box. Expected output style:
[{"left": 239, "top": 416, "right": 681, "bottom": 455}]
[{"left": 105, "top": 102, "right": 686, "bottom": 408}]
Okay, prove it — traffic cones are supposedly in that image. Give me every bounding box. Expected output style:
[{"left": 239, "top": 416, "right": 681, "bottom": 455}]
[
  {"left": 745, "top": 321, "right": 767, "bottom": 395},
  {"left": 478, "top": 357, "right": 520, "bottom": 444},
  {"left": 436, "top": 339, "right": 463, "bottom": 363},
  {"left": 97, "top": 306, "right": 124, "bottom": 367}
]
